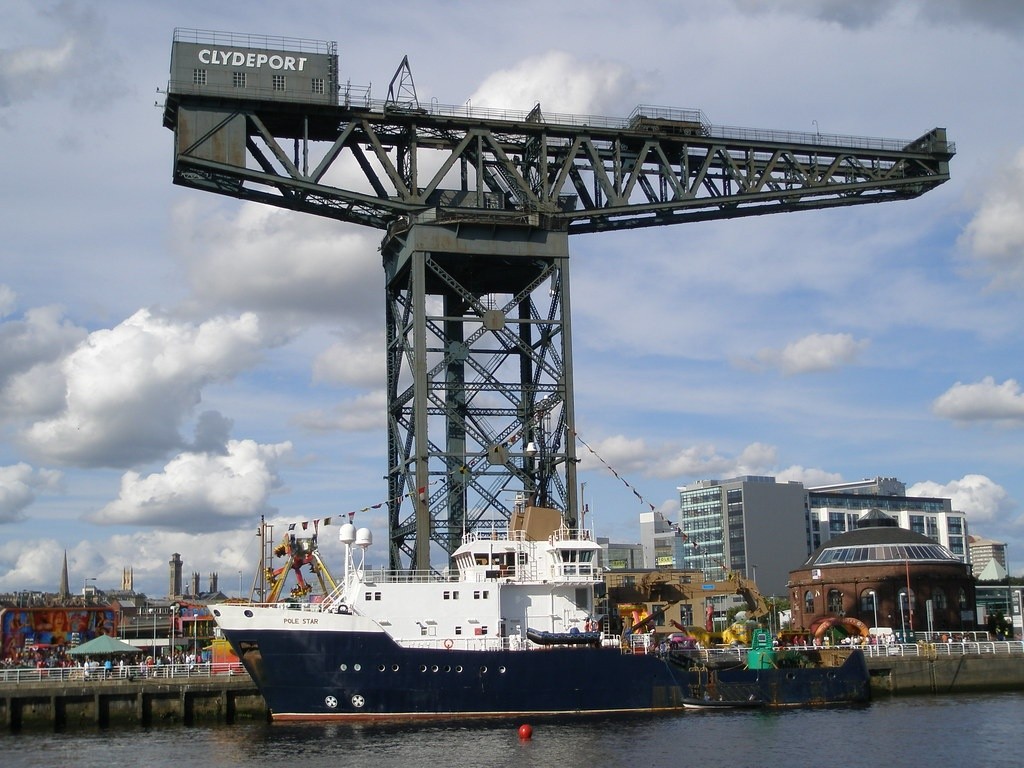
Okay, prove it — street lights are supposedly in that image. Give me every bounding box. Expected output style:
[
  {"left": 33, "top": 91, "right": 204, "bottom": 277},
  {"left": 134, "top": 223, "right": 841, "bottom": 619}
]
[
  {"left": 193, "top": 613, "right": 198, "bottom": 664},
  {"left": 868, "top": 590, "right": 877, "bottom": 628},
  {"left": 899, "top": 592, "right": 906, "bottom": 643},
  {"left": 147, "top": 608, "right": 155, "bottom": 664},
  {"left": 1013, "top": 590, "right": 1023, "bottom": 640},
  {"left": 769, "top": 593, "right": 776, "bottom": 634},
  {"left": 170, "top": 605, "right": 176, "bottom": 680},
  {"left": 82, "top": 577, "right": 98, "bottom": 608}
]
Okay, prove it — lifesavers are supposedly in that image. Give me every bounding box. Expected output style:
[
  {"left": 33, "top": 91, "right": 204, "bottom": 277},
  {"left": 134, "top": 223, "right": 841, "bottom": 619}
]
[
  {"left": 589, "top": 619, "right": 599, "bottom": 632},
  {"left": 443, "top": 639, "right": 454, "bottom": 648}
]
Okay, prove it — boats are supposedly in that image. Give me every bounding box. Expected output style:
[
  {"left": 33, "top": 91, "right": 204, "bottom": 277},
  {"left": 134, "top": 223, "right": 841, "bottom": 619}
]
[{"left": 200, "top": 522, "right": 872, "bottom": 727}]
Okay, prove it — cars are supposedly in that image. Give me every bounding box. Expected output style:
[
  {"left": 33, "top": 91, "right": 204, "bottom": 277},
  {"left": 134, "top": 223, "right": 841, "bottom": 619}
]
[{"left": 668, "top": 632, "right": 697, "bottom": 647}]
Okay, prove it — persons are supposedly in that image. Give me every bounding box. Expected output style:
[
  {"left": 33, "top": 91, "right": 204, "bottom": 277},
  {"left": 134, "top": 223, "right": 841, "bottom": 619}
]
[
  {"left": 3, "top": 649, "right": 131, "bottom": 680},
  {"left": 931, "top": 632, "right": 970, "bottom": 653},
  {"left": 776, "top": 636, "right": 822, "bottom": 649},
  {"left": 840, "top": 632, "right": 899, "bottom": 651},
  {"left": 139, "top": 650, "right": 213, "bottom": 679},
  {"left": 648, "top": 639, "right": 701, "bottom": 658},
  {"left": 625, "top": 626, "right": 634, "bottom": 649}
]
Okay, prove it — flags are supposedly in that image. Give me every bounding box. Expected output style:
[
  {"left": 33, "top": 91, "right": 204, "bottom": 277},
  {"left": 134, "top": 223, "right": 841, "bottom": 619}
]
[
  {"left": 568, "top": 427, "right": 774, "bottom": 608},
  {"left": 288, "top": 427, "right": 522, "bottom": 531}
]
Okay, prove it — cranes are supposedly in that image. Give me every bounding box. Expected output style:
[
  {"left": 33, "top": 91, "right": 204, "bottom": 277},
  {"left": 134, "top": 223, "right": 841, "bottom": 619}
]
[{"left": 153, "top": 29, "right": 957, "bottom": 582}]
